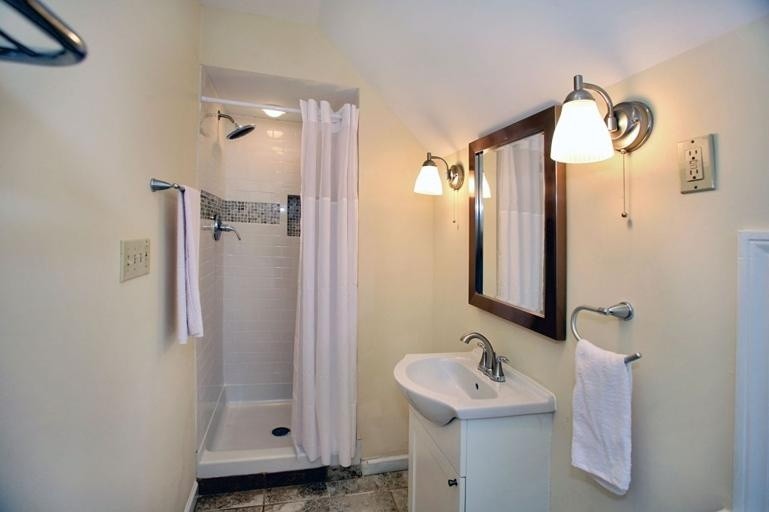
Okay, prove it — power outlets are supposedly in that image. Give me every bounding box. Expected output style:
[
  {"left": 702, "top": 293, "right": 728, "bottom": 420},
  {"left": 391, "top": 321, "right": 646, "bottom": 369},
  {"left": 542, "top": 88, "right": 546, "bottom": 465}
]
[{"left": 677, "top": 134, "right": 715, "bottom": 194}]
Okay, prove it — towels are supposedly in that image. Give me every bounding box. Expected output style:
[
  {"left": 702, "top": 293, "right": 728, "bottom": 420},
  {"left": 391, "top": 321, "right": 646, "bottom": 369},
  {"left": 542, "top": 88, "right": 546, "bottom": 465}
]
[
  {"left": 175, "top": 185, "right": 204, "bottom": 344},
  {"left": 567, "top": 340, "right": 637, "bottom": 497}
]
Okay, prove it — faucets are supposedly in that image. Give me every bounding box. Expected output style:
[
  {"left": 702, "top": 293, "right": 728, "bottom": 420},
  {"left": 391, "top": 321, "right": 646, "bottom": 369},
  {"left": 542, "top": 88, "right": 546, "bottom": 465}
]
[{"left": 459, "top": 331, "right": 511, "bottom": 382}]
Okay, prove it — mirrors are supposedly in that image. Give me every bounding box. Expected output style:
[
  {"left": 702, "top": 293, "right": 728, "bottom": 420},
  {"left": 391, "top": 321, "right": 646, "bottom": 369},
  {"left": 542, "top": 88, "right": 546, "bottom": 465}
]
[{"left": 469, "top": 105, "right": 567, "bottom": 342}]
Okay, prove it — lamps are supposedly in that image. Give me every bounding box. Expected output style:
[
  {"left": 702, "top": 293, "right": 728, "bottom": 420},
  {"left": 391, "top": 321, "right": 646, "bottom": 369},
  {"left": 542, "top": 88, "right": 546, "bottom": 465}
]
[
  {"left": 548, "top": 73, "right": 654, "bottom": 165},
  {"left": 414, "top": 151, "right": 464, "bottom": 196}
]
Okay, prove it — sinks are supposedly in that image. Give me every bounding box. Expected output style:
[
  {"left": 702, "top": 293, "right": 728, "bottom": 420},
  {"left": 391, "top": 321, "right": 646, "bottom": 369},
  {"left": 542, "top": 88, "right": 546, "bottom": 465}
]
[{"left": 394, "top": 348, "right": 501, "bottom": 410}]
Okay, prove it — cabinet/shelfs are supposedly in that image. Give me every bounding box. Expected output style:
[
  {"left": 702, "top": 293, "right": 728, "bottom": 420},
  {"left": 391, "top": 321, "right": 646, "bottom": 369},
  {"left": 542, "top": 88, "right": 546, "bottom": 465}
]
[{"left": 407, "top": 409, "right": 552, "bottom": 512}]
